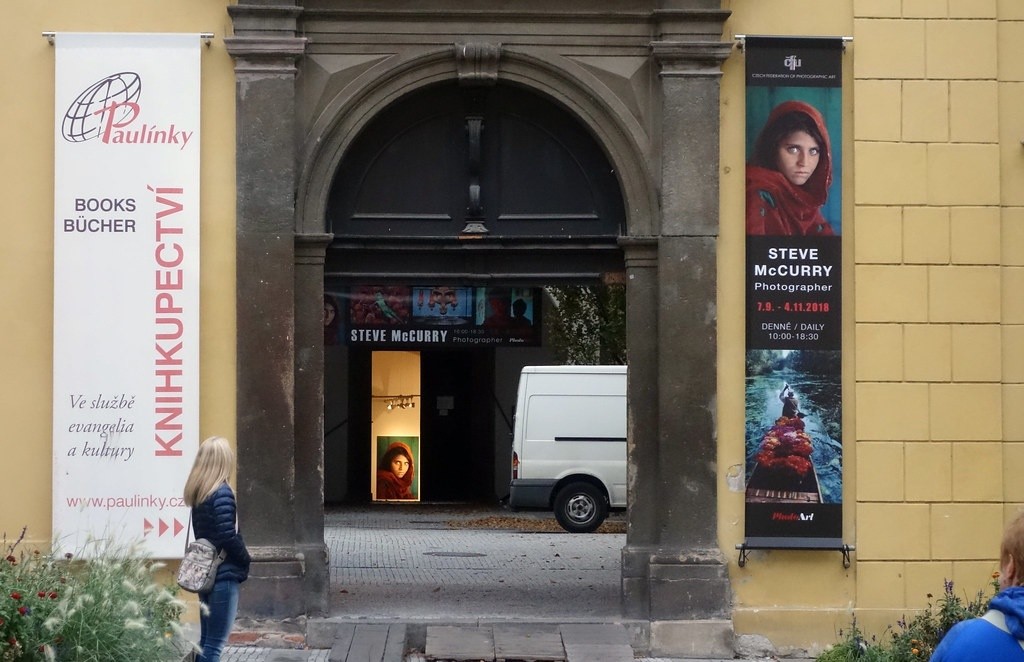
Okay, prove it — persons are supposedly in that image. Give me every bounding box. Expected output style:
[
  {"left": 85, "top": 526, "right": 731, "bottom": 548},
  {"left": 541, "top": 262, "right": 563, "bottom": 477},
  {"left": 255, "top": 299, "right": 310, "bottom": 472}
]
[
  {"left": 377, "top": 441, "right": 418, "bottom": 500},
  {"left": 925, "top": 514, "right": 1023, "bottom": 662},
  {"left": 745, "top": 102, "right": 837, "bottom": 234},
  {"left": 178, "top": 436, "right": 251, "bottom": 662}
]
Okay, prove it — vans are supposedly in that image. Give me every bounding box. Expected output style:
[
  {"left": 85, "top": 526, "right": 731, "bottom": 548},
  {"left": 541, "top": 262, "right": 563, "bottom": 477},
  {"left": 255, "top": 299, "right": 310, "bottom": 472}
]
[{"left": 509, "top": 364, "right": 628, "bottom": 532}]
